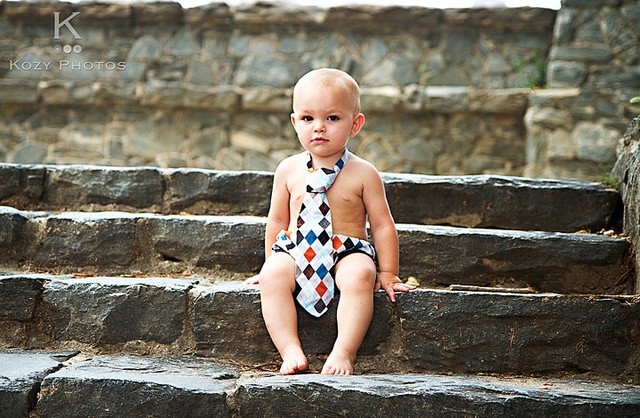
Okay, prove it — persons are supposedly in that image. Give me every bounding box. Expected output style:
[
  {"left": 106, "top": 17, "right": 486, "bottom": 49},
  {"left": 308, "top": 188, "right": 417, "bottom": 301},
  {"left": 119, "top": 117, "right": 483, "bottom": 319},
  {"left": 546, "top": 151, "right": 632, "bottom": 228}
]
[{"left": 246, "top": 67, "right": 416, "bottom": 377}]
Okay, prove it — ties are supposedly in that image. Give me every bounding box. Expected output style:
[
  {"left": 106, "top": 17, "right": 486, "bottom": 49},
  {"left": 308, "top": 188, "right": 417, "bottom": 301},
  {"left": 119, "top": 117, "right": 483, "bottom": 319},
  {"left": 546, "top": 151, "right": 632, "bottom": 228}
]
[{"left": 294, "top": 147, "right": 349, "bottom": 318}]
